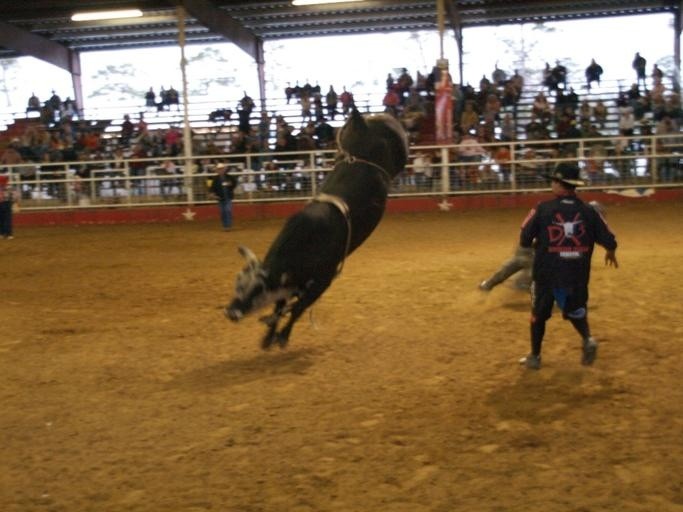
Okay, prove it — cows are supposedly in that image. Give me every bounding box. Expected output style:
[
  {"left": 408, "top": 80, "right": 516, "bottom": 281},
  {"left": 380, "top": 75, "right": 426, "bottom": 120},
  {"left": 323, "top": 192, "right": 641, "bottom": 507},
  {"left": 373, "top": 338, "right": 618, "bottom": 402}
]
[{"left": 221, "top": 103, "right": 411, "bottom": 350}]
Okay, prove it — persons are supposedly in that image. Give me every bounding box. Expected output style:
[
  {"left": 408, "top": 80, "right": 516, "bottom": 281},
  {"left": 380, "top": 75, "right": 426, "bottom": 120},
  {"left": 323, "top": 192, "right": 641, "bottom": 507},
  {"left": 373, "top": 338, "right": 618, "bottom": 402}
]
[
  {"left": 190, "top": 66, "right": 442, "bottom": 204},
  {"left": 517, "top": 163, "right": 618, "bottom": 369},
  {"left": 208, "top": 163, "right": 236, "bottom": 230},
  {"left": 0, "top": 176, "right": 16, "bottom": 241},
  {"left": 1, "top": 85, "right": 185, "bottom": 204},
  {"left": 449, "top": 52, "right": 683, "bottom": 187},
  {"left": 477, "top": 244, "right": 534, "bottom": 293}
]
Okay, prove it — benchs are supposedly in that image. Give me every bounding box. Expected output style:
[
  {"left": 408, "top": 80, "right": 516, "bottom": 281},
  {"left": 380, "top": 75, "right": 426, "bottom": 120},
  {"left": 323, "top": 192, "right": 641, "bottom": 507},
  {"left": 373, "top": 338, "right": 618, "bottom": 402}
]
[{"left": 0, "top": 75, "right": 683, "bottom": 211}]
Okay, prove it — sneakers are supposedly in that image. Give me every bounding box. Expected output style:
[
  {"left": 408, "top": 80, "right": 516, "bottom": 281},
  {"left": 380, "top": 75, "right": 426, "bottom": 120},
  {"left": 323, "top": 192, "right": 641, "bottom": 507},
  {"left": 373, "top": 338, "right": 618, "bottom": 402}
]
[
  {"left": 517, "top": 352, "right": 542, "bottom": 369},
  {"left": 581, "top": 336, "right": 597, "bottom": 366}
]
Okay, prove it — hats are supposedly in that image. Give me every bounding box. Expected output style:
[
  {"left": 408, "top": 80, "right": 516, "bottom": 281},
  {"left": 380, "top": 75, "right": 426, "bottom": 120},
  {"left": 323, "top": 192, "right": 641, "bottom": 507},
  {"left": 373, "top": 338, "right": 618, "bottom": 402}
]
[
  {"left": 538, "top": 159, "right": 588, "bottom": 189},
  {"left": 212, "top": 162, "right": 227, "bottom": 170}
]
